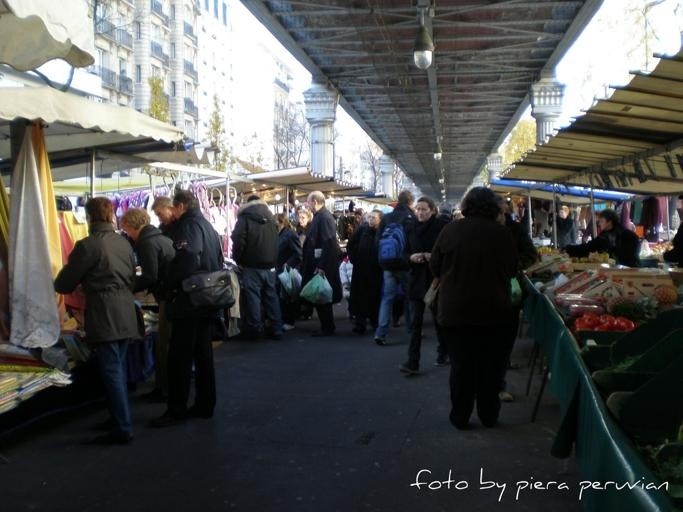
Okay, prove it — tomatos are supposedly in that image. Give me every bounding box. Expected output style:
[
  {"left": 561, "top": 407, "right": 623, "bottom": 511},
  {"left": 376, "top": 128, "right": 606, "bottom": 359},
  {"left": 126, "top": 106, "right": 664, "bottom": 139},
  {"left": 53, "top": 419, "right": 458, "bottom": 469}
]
[{"left": 569, "top": 311, "right": 640, "bottom": 330}]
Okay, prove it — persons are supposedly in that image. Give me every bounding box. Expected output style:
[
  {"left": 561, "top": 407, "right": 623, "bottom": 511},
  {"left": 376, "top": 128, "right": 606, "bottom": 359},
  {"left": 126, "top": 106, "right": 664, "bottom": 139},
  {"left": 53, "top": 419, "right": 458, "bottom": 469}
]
[
  {"left": 232, "top": 188, "right": 385, "bottom": 343},
  {"left": 397, "top": 197, "right": 454, "bottom": 376},
  {"left": 531, "top": 197, "right": 549, "bottom": 237},
  {"left": 114, "top": 208, "right": 182, "bottom": 408},
  {"left": 427, "top": 185, "right": 538, "bottom": 433},
  {"left": 549, "top": 205, "right": 576, "bottom": 250},
  {"left": 561, "top": 208, "right": 641, "bottom": 270},
  {"left": 149, "top": 195, "right": 181, "bottom": 239},
  {"left": 373, "top": 190, "right": 417, "bottom": 346},
  {"left": 51, "top": 193, "right": 144, "bottom": 450},
  {"left": 662, "top": 190, "right": 683, "bottom": 267},
  {"left": 431, "top": 200, "right": 465, "bottom": 223},
  {"left": 161, "top": 191, "right": 228, "bottom": 420}
]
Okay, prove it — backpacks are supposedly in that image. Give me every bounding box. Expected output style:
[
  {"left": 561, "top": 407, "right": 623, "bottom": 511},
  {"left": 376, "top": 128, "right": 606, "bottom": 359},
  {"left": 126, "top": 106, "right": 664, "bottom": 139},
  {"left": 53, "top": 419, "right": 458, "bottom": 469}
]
[{"left": 379, "top": 215, "right": 406, "bottom": 265}]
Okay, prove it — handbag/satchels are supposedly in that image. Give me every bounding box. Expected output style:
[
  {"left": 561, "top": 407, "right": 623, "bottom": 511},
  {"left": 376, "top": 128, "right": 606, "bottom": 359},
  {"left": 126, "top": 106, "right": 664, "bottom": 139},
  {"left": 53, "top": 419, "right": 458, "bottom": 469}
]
[{"left": 182, "top": 269, "right": 235, "bottom": 313}]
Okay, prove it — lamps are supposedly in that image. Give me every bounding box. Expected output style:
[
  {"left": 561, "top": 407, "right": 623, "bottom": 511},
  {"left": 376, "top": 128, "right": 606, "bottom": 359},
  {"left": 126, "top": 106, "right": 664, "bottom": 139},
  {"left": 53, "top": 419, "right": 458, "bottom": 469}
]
[
  {"left": 438, "top": 173, "right": 446, "bottom": 203},
  {"left": 411, "top": 7, "right": 435, "bottom": 69},
  {"left": 430, "top": 135, "right": 443, "bottom": 161}
]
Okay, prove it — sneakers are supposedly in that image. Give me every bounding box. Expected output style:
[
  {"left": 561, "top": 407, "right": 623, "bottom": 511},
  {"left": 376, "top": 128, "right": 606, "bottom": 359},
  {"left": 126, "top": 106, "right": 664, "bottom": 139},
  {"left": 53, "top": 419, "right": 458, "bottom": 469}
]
[
  {"left": 373, "top": 335, "right": 386, "bottom": 345},
  {"left": 270, "top": 334, "right": 282, "bottom": 341},
  {"left": 312, "top": 327, "right": 336, "bottom": 336},
  {"left": 282, "top": 323, "right": 295, "bottom": 331},
  {"left": 393, "top": 319, "right": 400, "bottom": 326},
  {"left": 432, "top": 360, "right": 450, "bottom": 366},
  {"left": 400, "top": 360, "right": 421, "bottom": 376},
  {"left": 498, "top": 391, "right": 515, "bottom": 405},
  {"left": 237, "top": 330, "right": 258, "bottom": 340},
  {"left": 352, "top": 327, "right": 367, "bottom": 336},
  {"left": 298, "top": 314, "right": 312, "bottom": 321}
]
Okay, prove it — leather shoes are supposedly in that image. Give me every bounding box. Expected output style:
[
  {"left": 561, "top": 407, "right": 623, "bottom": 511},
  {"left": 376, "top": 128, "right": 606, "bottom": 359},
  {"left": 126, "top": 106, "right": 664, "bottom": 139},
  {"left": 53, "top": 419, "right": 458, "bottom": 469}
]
[
  {"left": 186, "top": 403, "right": 215, "bottom": 418},
  {"left": 96, "top": 430, "right": 135, "bottom": 446},
  {"left": 150, "top": 413, "right": 185, "bottom": 428}
]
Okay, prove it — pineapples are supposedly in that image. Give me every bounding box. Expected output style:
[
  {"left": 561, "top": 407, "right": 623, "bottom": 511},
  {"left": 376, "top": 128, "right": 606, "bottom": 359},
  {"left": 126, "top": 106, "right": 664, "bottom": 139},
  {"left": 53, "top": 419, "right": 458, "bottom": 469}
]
[
  {"left": 654, "top": 282, "right": 683, "bottom": 303},
  {"left": 607, "top": 296, "right": 659, "bottom": 321}
]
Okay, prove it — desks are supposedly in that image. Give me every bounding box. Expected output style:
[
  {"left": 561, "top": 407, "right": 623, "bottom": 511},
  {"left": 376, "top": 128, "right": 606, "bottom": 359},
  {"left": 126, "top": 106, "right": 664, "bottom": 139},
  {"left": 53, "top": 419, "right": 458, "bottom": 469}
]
[{"left": 518, "top": 267, "right": 681, "bottom": 512}]
